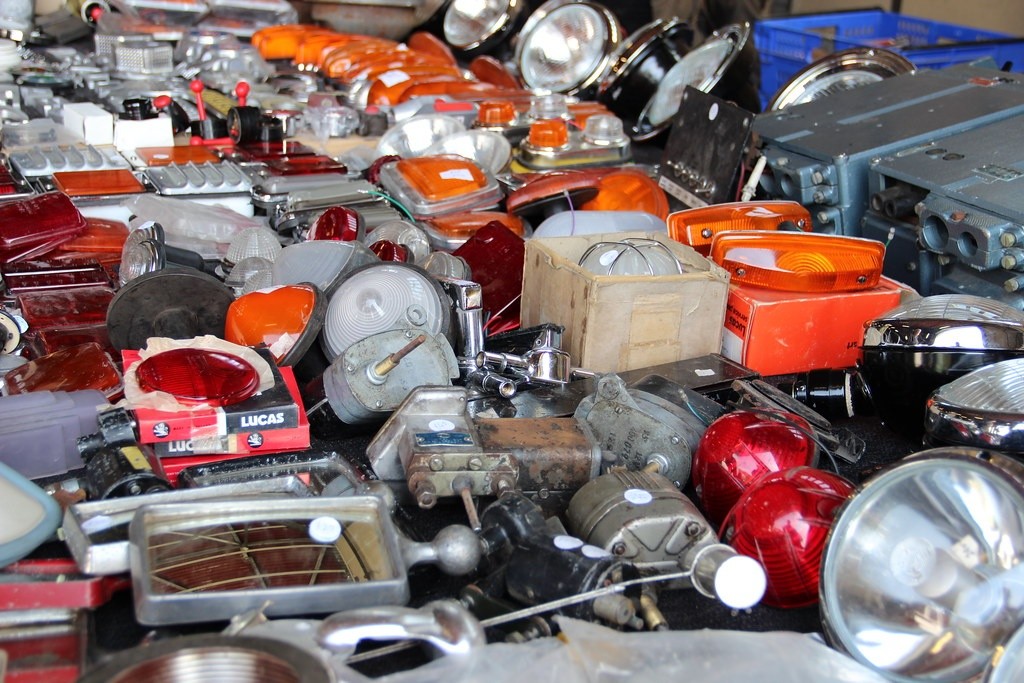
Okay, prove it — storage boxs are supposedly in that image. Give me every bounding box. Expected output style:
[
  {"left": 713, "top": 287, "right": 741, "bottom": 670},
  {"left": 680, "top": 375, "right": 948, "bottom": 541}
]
[
  {"left": 464, "top": 351, "right": 763, "bottom": 419},
  {"left": 721, "top": 276, "right": 903, "bottom": 377},
  {"left": 153, "top": 365, "right": 310, "bottom": 460},
  {"left": 756, "top": 11, "right": 1024, "bottom": 115},
  {"left": 514, "top": 228, "right": 732, "bottom": 374},
  {"left": 117, "top": 343, "right": 300, "bottom": 443}
]
